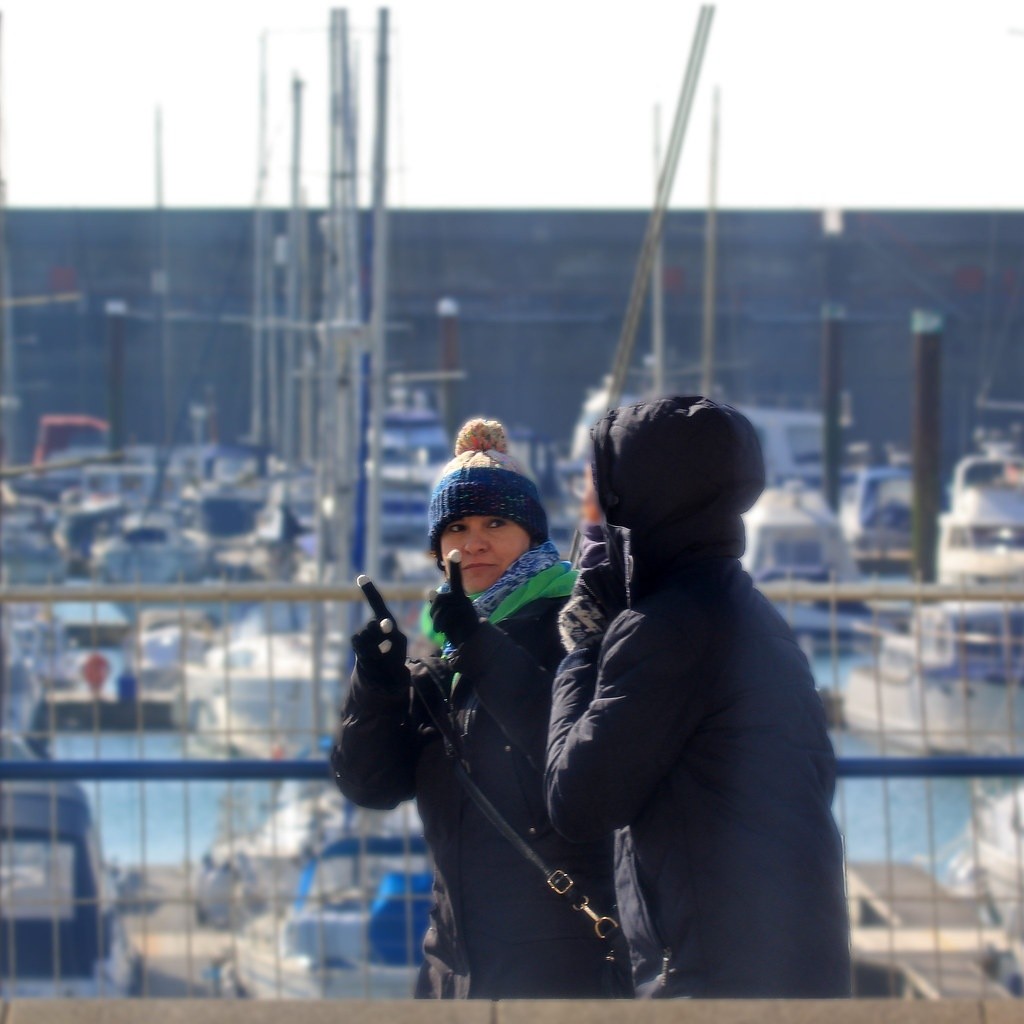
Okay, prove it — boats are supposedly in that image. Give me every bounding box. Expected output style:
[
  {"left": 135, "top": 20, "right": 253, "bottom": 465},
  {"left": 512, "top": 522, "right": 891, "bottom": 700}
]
[
  {"left": 836, "top": 601, "right": 1022, "bottom": 761},
  {"left": 744, "top": 455, "right": 1024, "bottom": 654},
  {"left": 105, "top": 736, "right": 441, "bottom": 998}
]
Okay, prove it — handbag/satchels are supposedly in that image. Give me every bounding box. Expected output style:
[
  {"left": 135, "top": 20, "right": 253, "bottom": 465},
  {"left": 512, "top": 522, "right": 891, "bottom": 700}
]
[{"left": 597, "top": 904, "right": 637, "bottom": 997}]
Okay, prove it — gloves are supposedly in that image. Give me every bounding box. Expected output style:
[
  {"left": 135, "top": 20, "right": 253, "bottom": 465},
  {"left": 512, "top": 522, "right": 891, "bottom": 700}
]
[
  {"left": 555, "top": 559, "right": 619, "bottom": 652},
  {"left": 350, "top": 574, "right": 408, "bottom": 693},
  {"left": 429, "top": 548, "right": 479, "bottom": 649}
]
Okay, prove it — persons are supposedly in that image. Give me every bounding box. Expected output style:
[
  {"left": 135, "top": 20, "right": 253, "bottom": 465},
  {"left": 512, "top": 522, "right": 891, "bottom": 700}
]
[
  {"left": 331, "top": 416, "right": 633, "bottom": 1001},
  {"left": 540, "top": 393, "right": 854, "bottom": 999}
]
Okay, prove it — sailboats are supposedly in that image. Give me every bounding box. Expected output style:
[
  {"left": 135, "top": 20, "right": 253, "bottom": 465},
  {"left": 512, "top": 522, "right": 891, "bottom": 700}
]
[{"left": 0, "top": 1, "right": 447, "bottom": 759}]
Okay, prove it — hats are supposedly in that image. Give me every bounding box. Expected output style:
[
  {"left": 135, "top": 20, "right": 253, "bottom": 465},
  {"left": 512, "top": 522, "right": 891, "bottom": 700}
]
[{"left": 428, "top": 418, "right": 550, "bottom": 571}]
